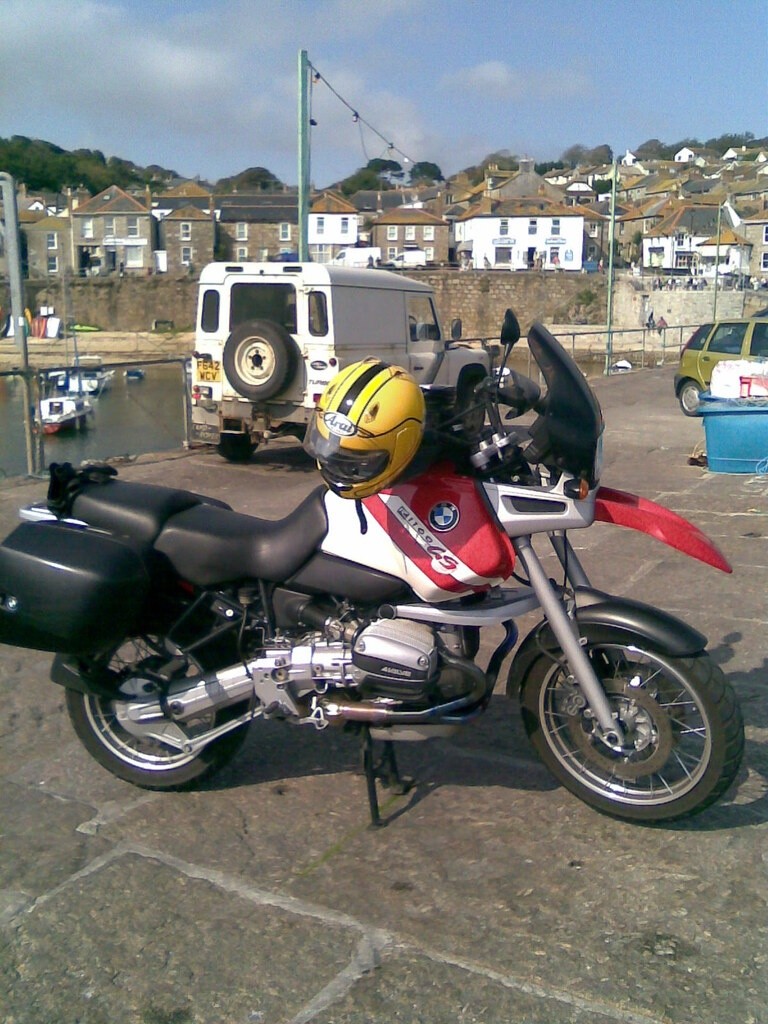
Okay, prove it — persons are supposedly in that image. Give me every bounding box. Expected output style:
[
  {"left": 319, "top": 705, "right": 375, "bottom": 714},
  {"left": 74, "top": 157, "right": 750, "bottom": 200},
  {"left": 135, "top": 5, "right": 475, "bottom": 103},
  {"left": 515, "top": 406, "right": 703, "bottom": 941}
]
[
  {"left": 631, "top": 260, "right": 635, "bottom": 270},
  {"left": 484, "top": 253, "right": 490, "bottom": 270},
  {"left": 652, "top": 276, "right": 766, "bottom": 292},
  {"left": 368, "top": 256, "right": 381, "bottom": 266},
  {"left": 600, "top": 257, "right": 605, "bottom": 275},
  {"left": 533, "top": 252, "right": 560, "bottom": 274},
  {"left": 83, "top": 249, "right": 90, "bottom": 264},
  {"left": 188, "top": 259, "right": 194, "bottom": 274},
  {"left": 648, "top": 312, "right": 667, "bottom": 336},
  {"left": 588, "top": 255, "right": 594, "bottom": 261}
]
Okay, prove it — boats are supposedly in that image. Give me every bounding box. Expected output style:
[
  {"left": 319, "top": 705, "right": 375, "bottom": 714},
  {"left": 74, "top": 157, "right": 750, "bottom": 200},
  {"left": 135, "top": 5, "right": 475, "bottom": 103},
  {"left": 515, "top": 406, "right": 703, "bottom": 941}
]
[
  {"left": 126, "top": 369, "right": 145, "bottom": 382},
  {"left": 65, "top": 358, "right": 115, "bottom": 394},
  {"left": 23, "top": 394, "right": 89, "bottom": 434}
]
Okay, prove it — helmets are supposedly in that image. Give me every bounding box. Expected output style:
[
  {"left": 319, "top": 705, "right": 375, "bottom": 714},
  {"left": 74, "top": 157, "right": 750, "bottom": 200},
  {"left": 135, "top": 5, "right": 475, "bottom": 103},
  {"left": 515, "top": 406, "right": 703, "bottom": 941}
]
[{"left": 317, "top": 356, "right": 427, "bottom": 499}]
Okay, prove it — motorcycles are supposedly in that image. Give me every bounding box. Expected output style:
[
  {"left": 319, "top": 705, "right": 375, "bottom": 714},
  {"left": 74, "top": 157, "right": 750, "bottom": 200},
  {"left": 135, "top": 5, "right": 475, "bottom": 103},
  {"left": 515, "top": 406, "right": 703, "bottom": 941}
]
[{"left": 0, "top": 310, "right": 747, "bottom": 834}]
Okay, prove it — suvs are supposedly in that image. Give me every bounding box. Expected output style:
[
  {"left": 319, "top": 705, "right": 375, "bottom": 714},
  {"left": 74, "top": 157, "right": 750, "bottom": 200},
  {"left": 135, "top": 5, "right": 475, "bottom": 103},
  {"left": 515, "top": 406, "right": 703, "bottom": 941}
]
[{"left": 182, "top": 262, "right": 487, "bottom": 460}]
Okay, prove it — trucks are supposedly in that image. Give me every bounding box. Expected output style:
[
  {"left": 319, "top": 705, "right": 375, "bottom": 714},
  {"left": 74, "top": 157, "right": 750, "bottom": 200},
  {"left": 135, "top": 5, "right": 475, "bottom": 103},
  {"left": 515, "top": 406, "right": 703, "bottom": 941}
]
[
  {"left": 387, "top": 251, "right": 426, "bottom": 271},
  {"left": 274, "top": 252, "right": 311, "bottom": 262},
  {"left": 330, "top": 247, "right": 381, "bottom": 267}
]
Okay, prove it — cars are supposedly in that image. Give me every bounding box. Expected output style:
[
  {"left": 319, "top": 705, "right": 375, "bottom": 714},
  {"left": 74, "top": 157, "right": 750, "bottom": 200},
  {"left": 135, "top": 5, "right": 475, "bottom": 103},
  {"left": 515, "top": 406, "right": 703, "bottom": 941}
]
[{"left": 673, "top": 317, "right": 768, "bottom": 417}]
